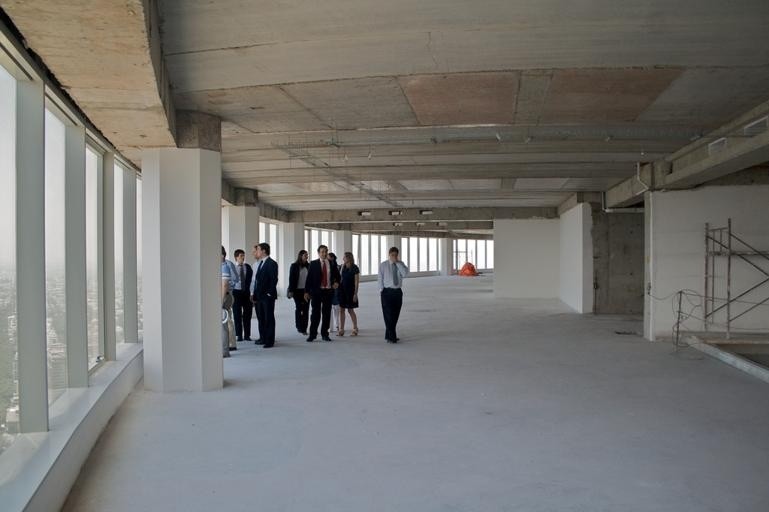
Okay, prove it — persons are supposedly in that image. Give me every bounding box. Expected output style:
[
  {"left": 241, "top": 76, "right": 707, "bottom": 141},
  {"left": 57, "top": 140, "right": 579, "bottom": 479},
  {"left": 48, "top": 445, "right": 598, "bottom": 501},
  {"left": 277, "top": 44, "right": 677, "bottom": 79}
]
[
  {"left": 220, "top": 242, "right": 360, "bottom": 349},
  {"left": 376, "top": 246, "right": 408, "bottom": 345}
]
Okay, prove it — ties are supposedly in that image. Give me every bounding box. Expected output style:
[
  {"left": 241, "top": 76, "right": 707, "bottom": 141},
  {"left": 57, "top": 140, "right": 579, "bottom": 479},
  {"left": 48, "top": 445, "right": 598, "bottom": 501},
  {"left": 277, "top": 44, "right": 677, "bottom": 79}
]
[
  {"left": 255, "top": 261, "right": 263, "bottom": 283},
  {"left": 392, "top": 263, "right": 399, "bottom": 286},
  {"left": 239, "top": 264, "right": 245, "bottom": 290},
  {"left": 322, "top": 260, "right": 327, "bottom": 288}
]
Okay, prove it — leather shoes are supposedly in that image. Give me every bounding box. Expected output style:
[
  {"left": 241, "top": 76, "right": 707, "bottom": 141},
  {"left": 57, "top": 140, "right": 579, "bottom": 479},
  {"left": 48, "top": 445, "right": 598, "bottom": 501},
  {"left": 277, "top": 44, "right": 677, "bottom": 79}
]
[
  {"left": 384, "top": 338, "right": 399, "bottom": 343},
  {"left": 223, "top": 336, "right": 251, "bottom": 358},
  {"left": 263, "top": 344, "right": 273, "bottom": 348},
  {"left": 298, "top": 328, "right": 358, "bottom": 341},
  {"left": 255, "top": 338, "right": 265, "bottom": 345}
]
